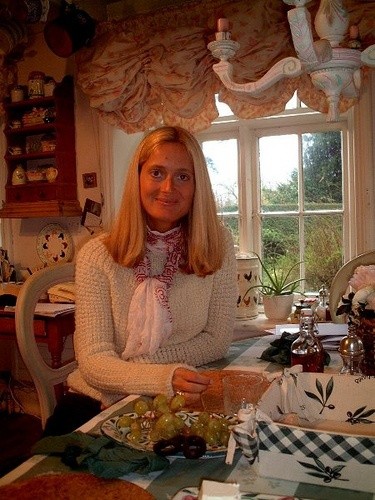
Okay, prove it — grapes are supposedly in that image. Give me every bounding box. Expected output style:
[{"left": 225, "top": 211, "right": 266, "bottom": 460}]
[{"left": 117, "top": 394, "right": 232, "bottom": 447}]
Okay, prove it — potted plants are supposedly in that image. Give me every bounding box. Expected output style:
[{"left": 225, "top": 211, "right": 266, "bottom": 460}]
[{"left": 241, "top": 250, "right": 314, "bottom": 321}]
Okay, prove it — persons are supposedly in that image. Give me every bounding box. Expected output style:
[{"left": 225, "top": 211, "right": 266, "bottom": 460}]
[{"left": 43, "top": 126, "right": 238, "bottom": 437}]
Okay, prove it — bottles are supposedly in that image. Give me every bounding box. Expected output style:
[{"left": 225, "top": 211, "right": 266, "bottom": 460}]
[
  {"left": 290, "top": 308, "right": 324, "bottom": 373},
  {"left": 11, "top": 163, "right": 26, "bottom": 186}
]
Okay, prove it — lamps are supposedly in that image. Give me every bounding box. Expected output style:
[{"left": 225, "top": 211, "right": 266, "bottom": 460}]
[{"left": 207, "top": 0, "right": 375, "bottom": 123}]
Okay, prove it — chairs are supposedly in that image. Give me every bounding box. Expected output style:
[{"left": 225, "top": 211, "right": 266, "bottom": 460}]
[{"left": 15, "top": 263, "right": 83, "bottom": 430}]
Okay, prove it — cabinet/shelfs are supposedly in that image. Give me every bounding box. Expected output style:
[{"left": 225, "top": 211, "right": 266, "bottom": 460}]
[{"left": 0, "top": 75, "right": 82, "bottom": 218}]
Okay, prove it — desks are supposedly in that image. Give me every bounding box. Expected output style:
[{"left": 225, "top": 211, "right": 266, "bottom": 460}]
[{"left": 0, "top": 320, "right": 375, "bottom": 500}]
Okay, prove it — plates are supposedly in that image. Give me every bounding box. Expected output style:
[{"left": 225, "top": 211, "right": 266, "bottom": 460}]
[
  {"left": 197, "top": 368, "right": 271, "bottom": 414},
  {"left": 36, "top": 223, "right": 73, "bottom": 267},
  {"left": 0, "top": 473, "right": 157, "bottom": 500},
  {"left": 101, "top": 410, "right": 239, "bottom": 460},
  {"left": 264, "top": 322, "right": 348, "bottom": 351}
]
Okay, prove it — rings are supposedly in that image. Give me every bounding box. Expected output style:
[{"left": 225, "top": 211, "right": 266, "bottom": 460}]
[{"left": 176, "top": 391, "right": 184, "bottom": 397}]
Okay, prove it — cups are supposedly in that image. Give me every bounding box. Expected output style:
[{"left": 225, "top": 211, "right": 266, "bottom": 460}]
[
  {"left": 8, "top": 146, "right": 24, "bottom": 155},
  {"left": 46, "top": 167, "right": 58, "bottom": 184},
  {"left": 221, "top": 374, "right": 264, "bottom": 417}
]
